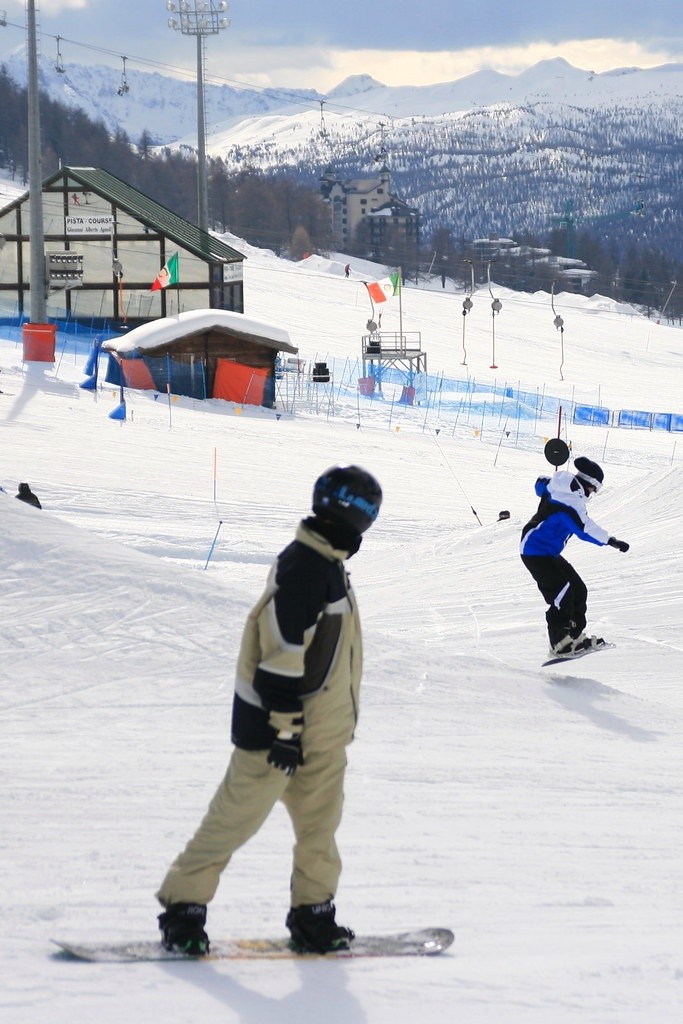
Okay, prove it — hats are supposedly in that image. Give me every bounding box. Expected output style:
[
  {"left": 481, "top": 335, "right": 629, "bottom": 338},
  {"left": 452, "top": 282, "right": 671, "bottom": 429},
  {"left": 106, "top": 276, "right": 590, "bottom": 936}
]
[{"left": 574, "top": 457, "right": 604, "bottom": 487}]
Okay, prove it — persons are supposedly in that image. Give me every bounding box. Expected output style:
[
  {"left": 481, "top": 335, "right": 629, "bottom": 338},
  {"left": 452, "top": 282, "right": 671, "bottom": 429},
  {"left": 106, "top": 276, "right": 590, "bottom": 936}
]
[
  {"left": 14, "top": 483, "right": 41, "bottom": 509},
  {"left": 374, "top": 148, "right": 386, "bottom": 161},
  {"left": 116, "top": 82, "right": 128, "bottom": 95},
  {"left": 345, "top": 264, "right": 350, "bottom": 278},
  {"left": 497, "top": 510, "right": 510, "bottom": 521},
  {"left": 630, "top": 201, "right": 640, "bottom": 214},
  {"left": 520, "top": 458, "right": 629, "bottom": 658},
  {"left": 153, "top": 464, "right": 382, "bottom": 955}
]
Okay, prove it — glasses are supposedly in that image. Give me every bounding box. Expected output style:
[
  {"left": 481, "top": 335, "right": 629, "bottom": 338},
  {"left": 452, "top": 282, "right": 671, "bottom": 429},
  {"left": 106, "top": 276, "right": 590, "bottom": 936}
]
[{"left": 596, "top": 483, "right": 602, "bottom": 492}]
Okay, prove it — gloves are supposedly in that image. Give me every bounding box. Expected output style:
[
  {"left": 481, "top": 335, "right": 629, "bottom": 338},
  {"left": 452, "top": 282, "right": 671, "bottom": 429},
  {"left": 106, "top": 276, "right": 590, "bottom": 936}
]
[
  {"left": 608, "top": 537, "right": 629, "bottom": 552},
  {"left": 267, "top": 730, "right": 303, "bottom": 776}
]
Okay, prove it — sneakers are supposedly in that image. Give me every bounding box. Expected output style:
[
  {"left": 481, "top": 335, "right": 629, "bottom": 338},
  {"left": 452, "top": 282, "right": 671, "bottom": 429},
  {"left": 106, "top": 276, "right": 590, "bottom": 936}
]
[
  {"left": 157, "top": 901, "right": 209, "bottom": 954},
  {"left": 571, "top": 631, "right": 604, "bottom": 648},
  {"left": 552, "top": 635, "right": 589, "bottom": 654},
  {"left": 285, "top": 900, "right": 355, "bottom": 947}
]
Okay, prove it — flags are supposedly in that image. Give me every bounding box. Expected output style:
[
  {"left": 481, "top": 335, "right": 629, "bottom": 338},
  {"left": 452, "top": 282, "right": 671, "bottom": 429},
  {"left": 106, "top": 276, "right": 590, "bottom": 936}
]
[
  {"left": 151, "top": 252, "right": 178, "bottom": 291},
  {"left": 368, "top": 271, "right": 399, "bottom": 304}
]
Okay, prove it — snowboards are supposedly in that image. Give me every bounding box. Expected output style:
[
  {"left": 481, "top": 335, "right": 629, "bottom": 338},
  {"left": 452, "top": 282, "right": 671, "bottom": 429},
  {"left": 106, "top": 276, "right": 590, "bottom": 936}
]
[
  {"left": 60, "top": 926, "right": 455, "bottom": 964},
  {"left": 540, "top": 641, "right": 617, "bottom": 668}
]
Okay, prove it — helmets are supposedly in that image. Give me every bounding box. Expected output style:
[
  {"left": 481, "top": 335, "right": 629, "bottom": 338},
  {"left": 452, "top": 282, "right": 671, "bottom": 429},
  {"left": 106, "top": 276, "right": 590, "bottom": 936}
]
[{"left": 313, "top": 465, "right": 383, "bottom": 533}]
